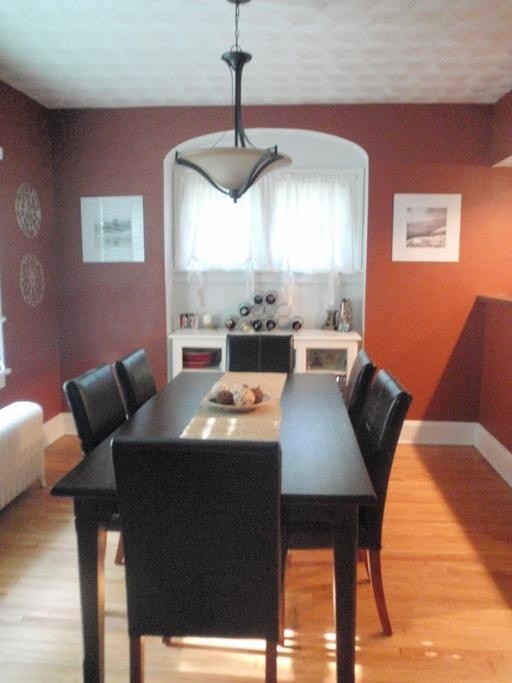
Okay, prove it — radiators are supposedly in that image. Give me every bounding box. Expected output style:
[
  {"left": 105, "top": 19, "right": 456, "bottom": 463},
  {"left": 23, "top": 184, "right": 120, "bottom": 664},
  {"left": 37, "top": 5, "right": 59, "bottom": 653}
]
[{"left": 0, "top": 400, "right": 46, "bottom": 511}]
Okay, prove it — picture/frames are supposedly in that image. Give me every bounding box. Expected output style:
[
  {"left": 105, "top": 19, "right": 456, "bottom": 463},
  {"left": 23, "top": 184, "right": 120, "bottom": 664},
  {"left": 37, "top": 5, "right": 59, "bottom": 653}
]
[
  {"left": 391, "top": 193, "right": 461, "bottom": 263},
  {"left": 79, "top": 195, "right": 145, "bottom": 264}
]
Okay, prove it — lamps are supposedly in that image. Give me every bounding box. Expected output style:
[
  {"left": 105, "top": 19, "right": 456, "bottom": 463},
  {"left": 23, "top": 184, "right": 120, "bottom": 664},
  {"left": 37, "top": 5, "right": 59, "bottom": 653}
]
[{"left": 175, "top": 0, "right": 293, "bottom": 202}]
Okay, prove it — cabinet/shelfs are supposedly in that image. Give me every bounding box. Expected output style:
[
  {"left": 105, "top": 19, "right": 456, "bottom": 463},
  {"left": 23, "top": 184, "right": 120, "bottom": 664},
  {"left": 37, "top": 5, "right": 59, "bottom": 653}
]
[{"left": 168, "top": 328, "right": 362, "bottom": 382}]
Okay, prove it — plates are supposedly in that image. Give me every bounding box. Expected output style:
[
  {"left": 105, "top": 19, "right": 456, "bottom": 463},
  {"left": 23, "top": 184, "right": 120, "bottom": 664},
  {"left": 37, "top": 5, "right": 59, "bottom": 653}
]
[{"left": 205, "top": 388, "right": 272, "bottom": 413}]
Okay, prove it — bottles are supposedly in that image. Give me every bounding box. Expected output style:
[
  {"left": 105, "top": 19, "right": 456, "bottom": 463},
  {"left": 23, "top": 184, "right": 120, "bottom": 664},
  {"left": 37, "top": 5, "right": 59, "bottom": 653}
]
[{"left": 337, "top": 298, "right": 353, "bottom": 332}]
